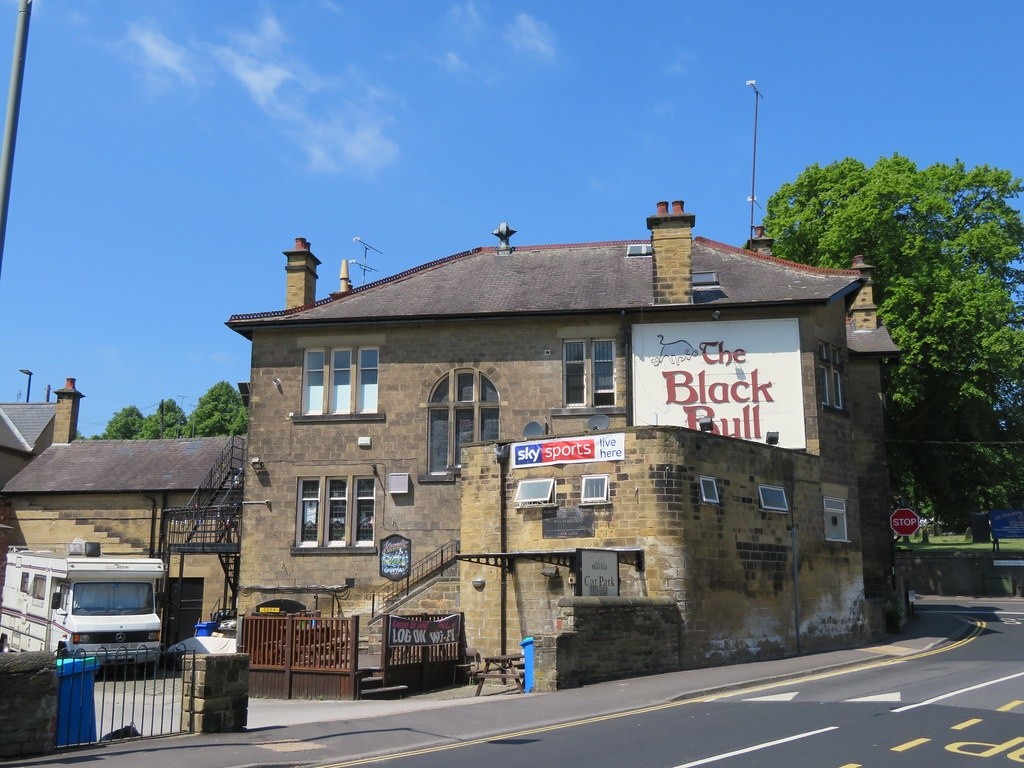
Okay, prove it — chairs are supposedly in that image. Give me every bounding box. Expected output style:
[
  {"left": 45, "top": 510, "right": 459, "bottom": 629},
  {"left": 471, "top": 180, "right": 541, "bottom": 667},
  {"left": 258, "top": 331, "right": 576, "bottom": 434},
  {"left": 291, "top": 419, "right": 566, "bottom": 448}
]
[{"left": 452, "top": 648, "right": 477, "bottom": 687}]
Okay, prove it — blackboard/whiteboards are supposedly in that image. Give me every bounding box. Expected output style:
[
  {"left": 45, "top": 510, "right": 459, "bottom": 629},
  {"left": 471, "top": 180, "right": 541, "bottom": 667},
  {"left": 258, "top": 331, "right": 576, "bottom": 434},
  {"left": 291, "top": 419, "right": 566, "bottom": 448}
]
[{"left": 378, "top": 533, "right": 412, "bottom": 582}]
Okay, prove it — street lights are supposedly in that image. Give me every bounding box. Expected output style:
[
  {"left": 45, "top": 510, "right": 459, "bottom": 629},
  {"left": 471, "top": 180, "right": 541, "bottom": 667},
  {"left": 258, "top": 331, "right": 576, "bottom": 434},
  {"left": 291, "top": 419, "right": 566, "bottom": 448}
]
[{"left": 20, "top": 369, "right": 34, "bottom": 402}]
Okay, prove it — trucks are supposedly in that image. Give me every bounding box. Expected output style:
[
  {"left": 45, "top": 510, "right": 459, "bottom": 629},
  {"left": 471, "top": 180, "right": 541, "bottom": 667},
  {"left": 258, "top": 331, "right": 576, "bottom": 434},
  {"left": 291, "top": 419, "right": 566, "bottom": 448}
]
[{"left": 0, "top": 537, "right": 170, "bottom": 671}]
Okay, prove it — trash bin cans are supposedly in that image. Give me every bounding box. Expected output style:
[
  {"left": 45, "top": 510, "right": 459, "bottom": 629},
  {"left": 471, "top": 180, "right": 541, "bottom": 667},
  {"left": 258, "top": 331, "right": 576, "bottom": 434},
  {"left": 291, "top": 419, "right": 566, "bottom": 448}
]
[
  {"left": 194, "top": 621, "right": 215, "bottom": 637},
  {"left": 56, "top": 656, "right": 101, "bottom": 746},
  {"left": 520, "top": 637, "right": 534, "bottom": 692}
]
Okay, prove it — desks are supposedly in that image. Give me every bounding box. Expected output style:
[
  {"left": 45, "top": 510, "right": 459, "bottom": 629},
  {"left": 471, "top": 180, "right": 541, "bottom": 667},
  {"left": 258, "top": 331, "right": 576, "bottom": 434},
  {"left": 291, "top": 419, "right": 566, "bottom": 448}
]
[
  {"left": 279, "top": 625, "right": 332, "bottom": 662},
  {"left": 474, "top": 654, "right": 525, "bottom": 696}
]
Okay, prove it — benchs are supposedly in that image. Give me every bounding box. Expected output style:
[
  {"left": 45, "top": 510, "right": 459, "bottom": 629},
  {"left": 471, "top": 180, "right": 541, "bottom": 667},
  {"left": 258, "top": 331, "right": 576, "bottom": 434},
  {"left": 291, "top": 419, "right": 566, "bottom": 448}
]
[
  {"left": 466, "top": 664, "right": 528, "bottom": 679},
  {"left": 262, "top": 640, "right": 282, "bottom": 648},
  {"left": 295, "top": 640, "right": 351, "bottom": 650}
]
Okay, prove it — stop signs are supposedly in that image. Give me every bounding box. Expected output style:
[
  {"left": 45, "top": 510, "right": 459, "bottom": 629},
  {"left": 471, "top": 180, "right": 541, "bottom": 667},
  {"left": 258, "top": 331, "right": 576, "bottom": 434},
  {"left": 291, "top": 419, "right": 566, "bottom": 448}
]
[{"left": 891, "top": 508, "right": 920, "bottom": 536}]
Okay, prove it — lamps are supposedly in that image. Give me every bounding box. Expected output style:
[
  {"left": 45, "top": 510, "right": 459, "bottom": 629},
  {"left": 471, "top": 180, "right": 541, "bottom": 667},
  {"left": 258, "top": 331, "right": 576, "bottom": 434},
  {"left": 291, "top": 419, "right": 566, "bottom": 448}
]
[
  {"left": 699, "top": 418, "right": 715, "bottom": 431},
  {"left": 711, "top": 310, "right": 720, "bottom": 321},
  {"left": 766, "top": 431, "right": 779, "bottom": 444},
  {"left": 250, "top": 457, "right": 266, "bottom": 472},
  {"left": 539, "top": 567, "right": 561, "bottom": 577},
  {"left": 371, "top": 461, "right": 386, "bottom": 496}
]
